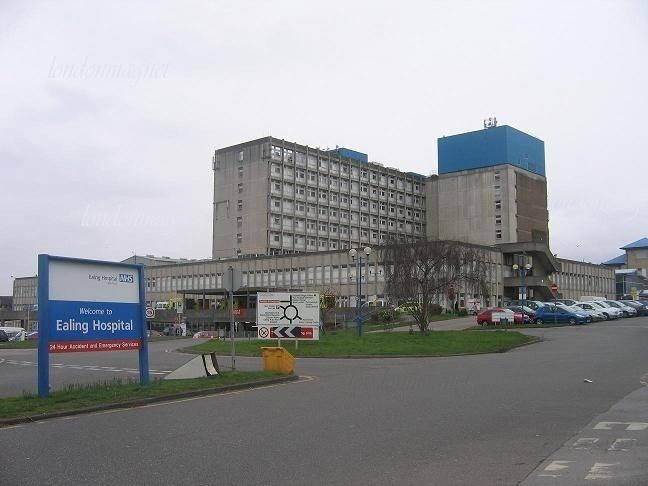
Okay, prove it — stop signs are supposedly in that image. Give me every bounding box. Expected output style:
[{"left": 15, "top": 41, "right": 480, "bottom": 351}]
[{"left": 551, "top": 284, "right": 558, "bottom": 292}]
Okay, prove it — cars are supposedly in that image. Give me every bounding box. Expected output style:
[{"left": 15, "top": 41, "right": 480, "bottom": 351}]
[
  {"left": 477, "top": 296, "right": 648, "bottom": 326},
  {"left": 147, "top": 327, "right": 219, "bottom": 338},
  {"left": 0, "top": 327, "right": 38, "bottom": 341},
  {"left": 357, "top": 299, "right": 423, "bottom": 314}
]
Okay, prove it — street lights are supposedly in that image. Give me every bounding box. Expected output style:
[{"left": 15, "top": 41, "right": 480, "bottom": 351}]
[
  {"left": 513, "top": 264, "right": 531, "bottom": 324},
  {"left": 349, "top": 247, "right": 371, "bottom": 336}
]
